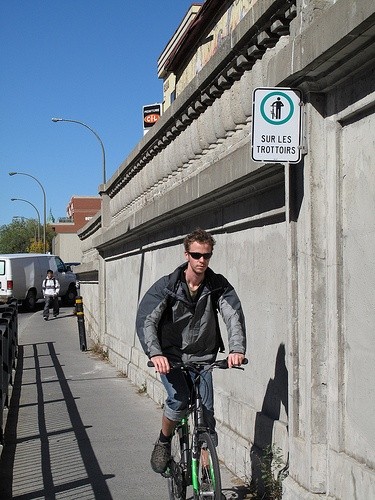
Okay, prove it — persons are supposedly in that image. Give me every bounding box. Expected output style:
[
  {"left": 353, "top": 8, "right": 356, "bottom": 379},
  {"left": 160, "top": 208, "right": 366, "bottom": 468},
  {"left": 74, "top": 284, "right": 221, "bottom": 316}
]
[
  {"left": 135, "top": 229, "right": 247, "bottom": 484},
  {"left": 41, "top": 269, "right": 61, "bottom": 321}
]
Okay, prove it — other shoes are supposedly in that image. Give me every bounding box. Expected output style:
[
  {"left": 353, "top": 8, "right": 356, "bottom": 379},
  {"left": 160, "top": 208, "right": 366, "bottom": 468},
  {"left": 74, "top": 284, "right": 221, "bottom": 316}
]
[
  {"left": 43, "top": 316, "right": 48, "bottom": 321},
  {"left": 53, "top": 315, "right": 57, "bottom": 318}
]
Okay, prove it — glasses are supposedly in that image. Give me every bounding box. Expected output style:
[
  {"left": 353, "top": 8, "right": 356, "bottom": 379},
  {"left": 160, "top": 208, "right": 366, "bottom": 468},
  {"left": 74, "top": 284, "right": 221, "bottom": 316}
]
[{"left": 187, "top": 250, "right": 212, "bottom": 259}]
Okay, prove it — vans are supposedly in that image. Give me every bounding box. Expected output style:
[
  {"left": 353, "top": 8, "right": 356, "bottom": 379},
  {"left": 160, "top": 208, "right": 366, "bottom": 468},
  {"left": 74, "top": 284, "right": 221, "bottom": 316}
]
[{"left": 0, "top": 253, "right": 78, "bottom": 312}]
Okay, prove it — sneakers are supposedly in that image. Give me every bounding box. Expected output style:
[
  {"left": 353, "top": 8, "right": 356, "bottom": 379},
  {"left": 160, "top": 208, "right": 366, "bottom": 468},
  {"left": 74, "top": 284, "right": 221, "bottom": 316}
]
[{"left": 150, "top": 433, "right": 172, "bottom": 474}]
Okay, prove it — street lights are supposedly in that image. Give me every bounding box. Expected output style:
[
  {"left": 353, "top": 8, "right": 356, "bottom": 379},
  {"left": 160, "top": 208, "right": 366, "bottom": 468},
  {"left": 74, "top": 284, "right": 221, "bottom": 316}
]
[
  {"left": 50, "top": 117, "right": 105, "bottom": 191},
  {"left": 13, "top": 216, "right": 37, "bottom": 243},
  {"left": 9, "top": 172, "right": 46, "bottom": 253},
  {"left": 11, "top": 197, "right": 41, "bottom": 243}
]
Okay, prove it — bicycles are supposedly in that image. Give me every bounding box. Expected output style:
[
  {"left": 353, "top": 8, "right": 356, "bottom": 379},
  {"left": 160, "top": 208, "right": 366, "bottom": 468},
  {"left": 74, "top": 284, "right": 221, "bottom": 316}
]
[{"left": 146, "top": 358, "right": 248, "bottom": 500}]
